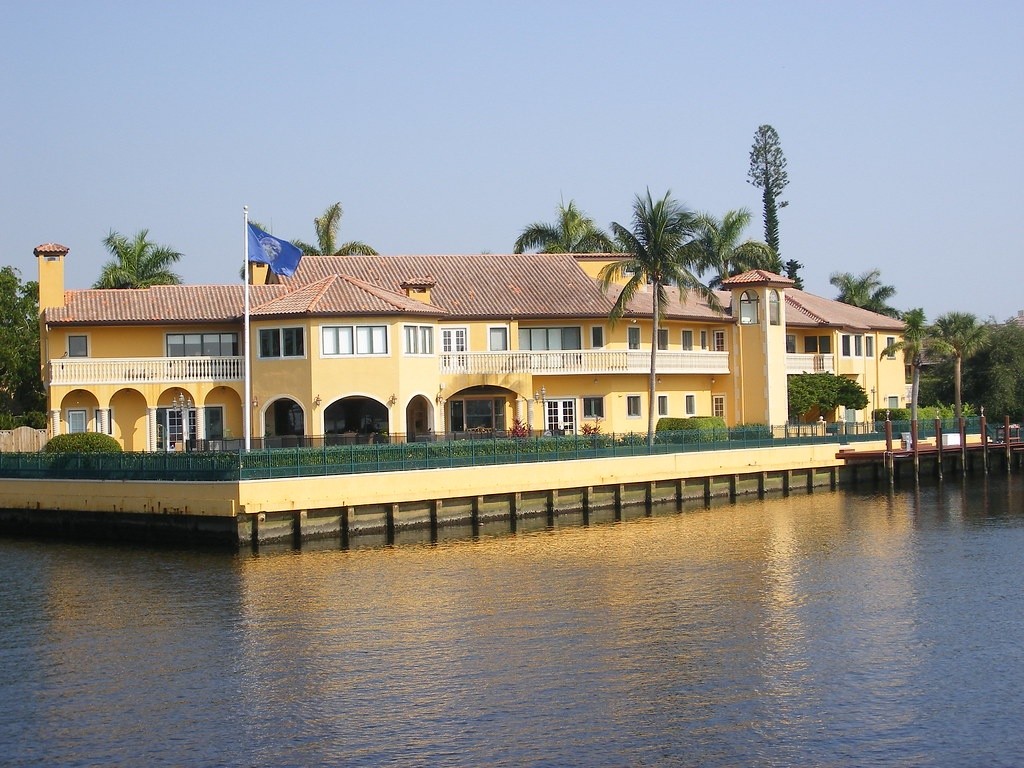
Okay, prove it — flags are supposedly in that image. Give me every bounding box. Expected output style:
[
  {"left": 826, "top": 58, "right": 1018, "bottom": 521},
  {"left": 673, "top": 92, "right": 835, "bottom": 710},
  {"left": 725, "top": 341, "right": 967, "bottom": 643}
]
[{"left": 248, "top": 221, "right": 302, "bottom": 277}]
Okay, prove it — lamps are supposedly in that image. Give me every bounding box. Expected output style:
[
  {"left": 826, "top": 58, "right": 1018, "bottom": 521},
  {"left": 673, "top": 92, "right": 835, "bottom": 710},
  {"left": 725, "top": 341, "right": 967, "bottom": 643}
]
[
  {"left": 594, "top": 375, "right": 598, "bottom": 384},
  {"left": 658, "top": 378, "right": 662, "bottom": 384},
  {"left": 389, "top": 393, "right": 397, "bottom": 406},
  {"left": 710, "top": 376, "right": 715, "bottom": 384},
  {"left": 314, "top": 394, "right": 322, "bottom": 406},
  {"left": 884, "top": 396, "right": 888, "bottom": 402},
  {"left": 252, "top": 396, "right": 258, "bottom": 408},
  {"left": 901, "top": 394, "right": 906, "bottom": 402},
  {"left": 79, "top": 390, "right": 83, "bottom": 397},
  {"left": 534, "top": 390, "right": 539, "bottom": 404},
  {"left": 436, "top": 392, "right": 443, "bottom": 404},
  {"left": 126, "top": 389, "right": 130, "bottom": 394}
]
